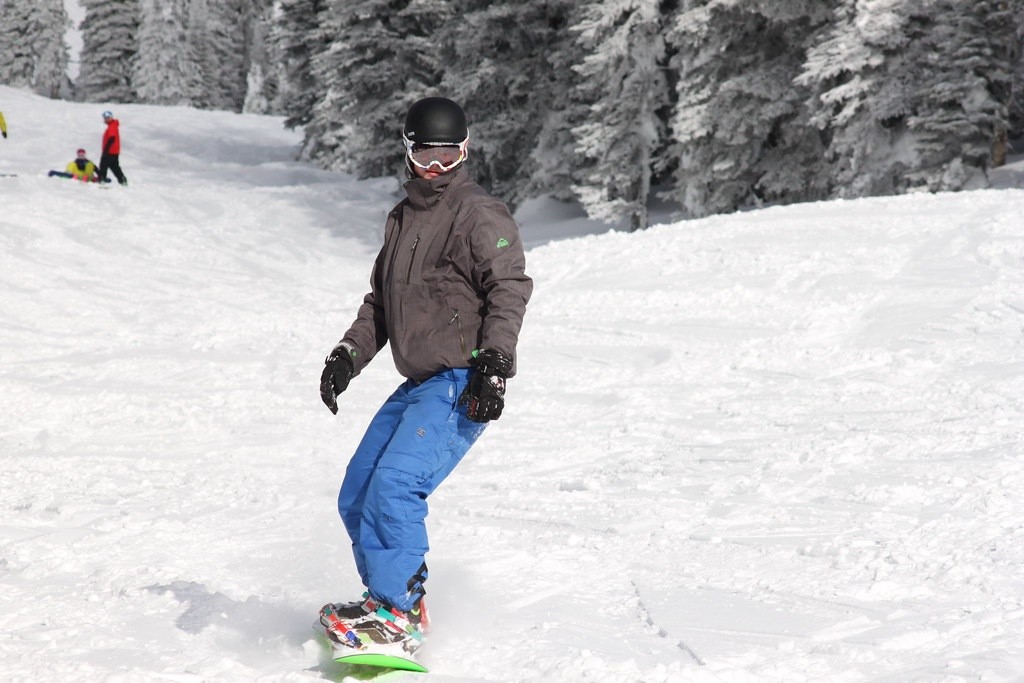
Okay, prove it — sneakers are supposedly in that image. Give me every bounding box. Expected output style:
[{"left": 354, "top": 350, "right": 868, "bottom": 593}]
[{"left": 318, "top": 596, "right": 427, "bottom": 653}]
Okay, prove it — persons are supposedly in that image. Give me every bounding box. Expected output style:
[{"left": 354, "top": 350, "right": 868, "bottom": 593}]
[
  {"left": 0, "top": 112, "right": 7, "bottom": 139},
  {"left": 66, "top": 149, "right": 99, "bottom": 183},
  {"left": 319, "top": 98, "right": 533, "bottom": 655},
  {"left": 98, "top": 111, "right": 127, "bottom": 184}
]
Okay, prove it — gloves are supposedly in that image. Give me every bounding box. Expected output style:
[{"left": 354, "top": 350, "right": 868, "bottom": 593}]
[
  {"left": 3, "top": 132, "right": 7, "bottom": 138},
  {"left": 318, "top": 347, "right": 352, "bottom": 414},
  {"left": 459, "top": 349, "right": 511, "bottom": 421}
]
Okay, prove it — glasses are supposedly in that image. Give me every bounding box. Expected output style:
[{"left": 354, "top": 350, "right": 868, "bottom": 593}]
[{"left": 404, "top": 139, "right": 468, "bottom": 171}]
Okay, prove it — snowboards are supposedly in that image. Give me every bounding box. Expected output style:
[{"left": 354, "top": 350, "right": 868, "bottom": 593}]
[{"left": 321, "top": 593, "right": 430, "bottom": 671}]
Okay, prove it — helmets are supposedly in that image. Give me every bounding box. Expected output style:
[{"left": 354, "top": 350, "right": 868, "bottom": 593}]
[
  {"left": 403, "top": 95, "right": 469, "bottom": 146},
  {"left": 77, "top": 149, "right": 86, "bottom": 154},
  {"left": 103, "top": 111, "right": 112, "bottom": 117}
]
